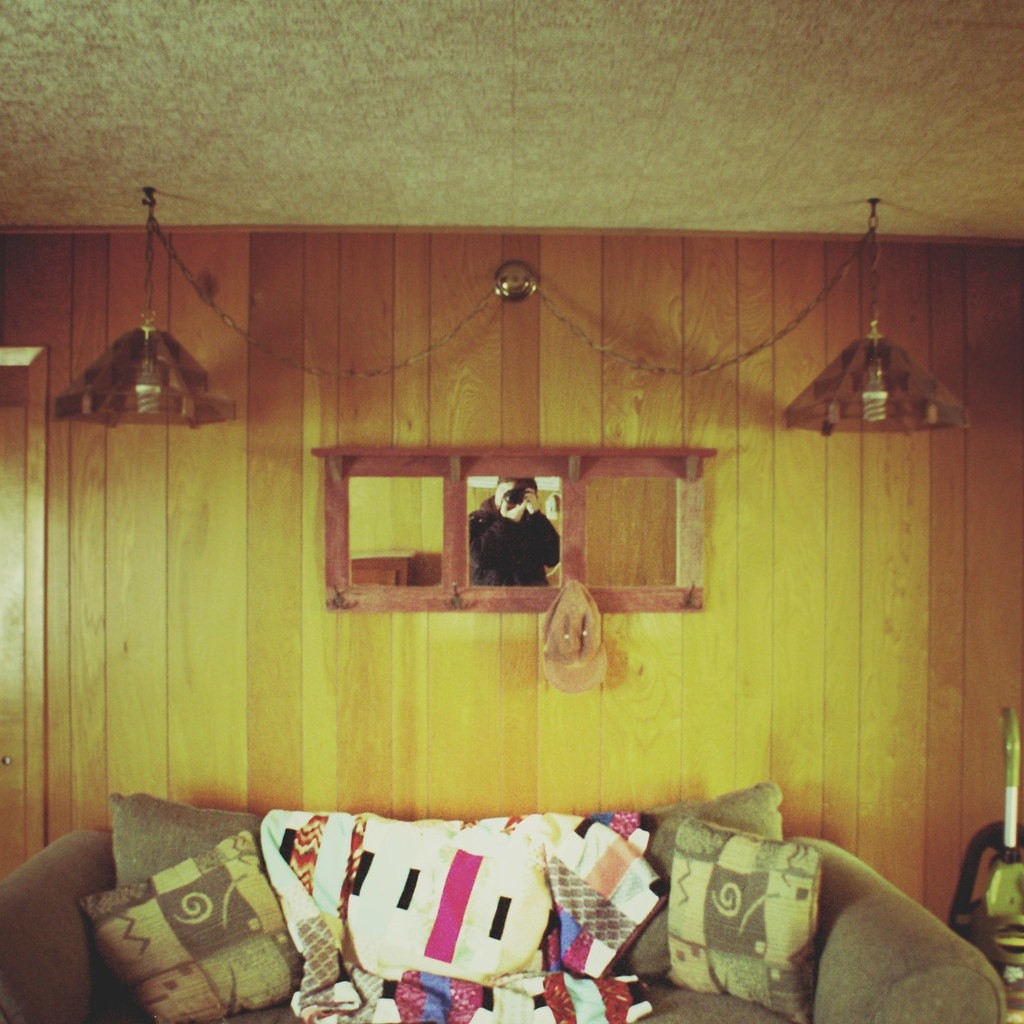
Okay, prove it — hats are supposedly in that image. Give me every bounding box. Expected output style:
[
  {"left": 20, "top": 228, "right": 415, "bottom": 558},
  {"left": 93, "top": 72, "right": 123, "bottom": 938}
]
[{"left": 541, "top": 581, "right": 607, "bottom": 693}]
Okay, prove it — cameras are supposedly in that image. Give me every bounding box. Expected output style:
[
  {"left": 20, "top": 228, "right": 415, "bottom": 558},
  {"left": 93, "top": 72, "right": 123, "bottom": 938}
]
[{"left": 503, "top": 489, "right": 527, "bottom": 505}]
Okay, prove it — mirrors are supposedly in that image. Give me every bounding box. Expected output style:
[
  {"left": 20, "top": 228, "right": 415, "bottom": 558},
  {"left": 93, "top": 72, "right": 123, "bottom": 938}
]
[{"left": 311, "top": 448, "right": 719, "bottom": 616}]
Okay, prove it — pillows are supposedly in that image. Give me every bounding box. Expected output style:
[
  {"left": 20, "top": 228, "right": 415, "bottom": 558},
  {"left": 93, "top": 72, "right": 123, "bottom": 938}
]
[
  {"left": 665, "top": 813, "right": 826, "bottom": 1024},
  {"left": 109, "top": 791, "right": 261, "bottom": 891},
  {"left": 78, "top": 831, "right": 305, "bottom": 1024},
  {"left": 623, "top": 783, "right": 784, "bottom": 977}
]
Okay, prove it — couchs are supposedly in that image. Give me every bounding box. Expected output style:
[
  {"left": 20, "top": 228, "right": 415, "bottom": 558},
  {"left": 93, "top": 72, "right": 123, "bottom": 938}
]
[{"left": 0, "top": 810, "right": 1006, "bottom": 1024}]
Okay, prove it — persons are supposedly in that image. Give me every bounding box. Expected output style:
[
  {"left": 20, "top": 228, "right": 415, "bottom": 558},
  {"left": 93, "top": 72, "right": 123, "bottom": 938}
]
[{"left": 467, "top": 476, "right": 560, "bottom": 586}]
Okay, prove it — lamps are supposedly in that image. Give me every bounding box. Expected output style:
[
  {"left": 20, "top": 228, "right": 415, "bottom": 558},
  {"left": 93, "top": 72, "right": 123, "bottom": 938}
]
[
  {"left": 782, "top": 199, "right": 967, "bottom": 438},
  {"left": 54, "top": 186, "right": 237, "bottom": 429}
]
